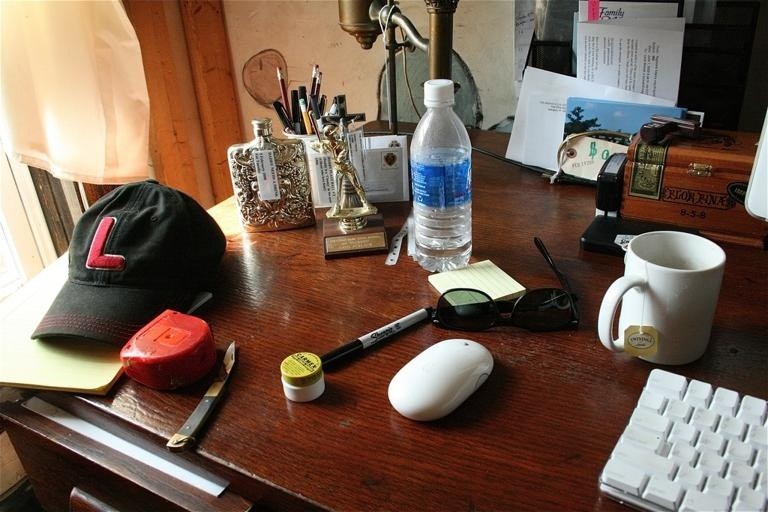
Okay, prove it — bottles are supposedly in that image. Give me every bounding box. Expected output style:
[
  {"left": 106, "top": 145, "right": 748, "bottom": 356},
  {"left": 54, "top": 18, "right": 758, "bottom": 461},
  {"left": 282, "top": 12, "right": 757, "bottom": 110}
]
[
  {"left": 409, "top": 79, "right": 474, "bottom": 274},
  {"left": 226, "top": 117, "right": 317, "bottom": 234}
]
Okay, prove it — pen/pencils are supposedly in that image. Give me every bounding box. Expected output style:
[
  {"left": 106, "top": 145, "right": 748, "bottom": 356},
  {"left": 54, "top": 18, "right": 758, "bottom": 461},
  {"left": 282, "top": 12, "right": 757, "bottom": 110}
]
[{"left": 273, "top": 64, "right": 328, "bottom": 142}]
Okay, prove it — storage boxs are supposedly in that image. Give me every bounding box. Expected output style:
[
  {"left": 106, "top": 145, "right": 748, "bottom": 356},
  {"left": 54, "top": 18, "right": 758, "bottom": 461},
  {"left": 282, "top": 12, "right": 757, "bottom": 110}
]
[{"left": 621, "top": 126, "right": 768, "bottom": 249}]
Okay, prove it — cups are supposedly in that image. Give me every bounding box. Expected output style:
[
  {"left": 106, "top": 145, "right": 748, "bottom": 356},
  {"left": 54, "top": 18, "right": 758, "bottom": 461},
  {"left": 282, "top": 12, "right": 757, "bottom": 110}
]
[
  {"left": 281, "top": 125, "right": 330, "bottom": 209},
  {"left": 596, "top": 230, "right": 731, "bottom": 368}
]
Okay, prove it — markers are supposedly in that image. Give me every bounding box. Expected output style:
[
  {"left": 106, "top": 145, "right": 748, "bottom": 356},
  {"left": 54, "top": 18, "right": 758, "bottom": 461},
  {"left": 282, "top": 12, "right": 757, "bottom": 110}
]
[{"left": 321, "top": 306, "right": 431, "bottom": 365}]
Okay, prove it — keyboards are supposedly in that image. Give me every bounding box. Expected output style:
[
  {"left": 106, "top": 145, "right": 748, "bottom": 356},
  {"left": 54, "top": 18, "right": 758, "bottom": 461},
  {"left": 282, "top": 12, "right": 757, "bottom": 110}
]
[{"left": 596, "top": 368, "right": 768, "bottom": 512}]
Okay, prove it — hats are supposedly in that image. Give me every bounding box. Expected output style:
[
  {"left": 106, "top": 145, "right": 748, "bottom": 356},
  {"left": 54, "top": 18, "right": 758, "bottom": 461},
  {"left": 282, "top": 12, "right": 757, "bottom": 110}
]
[{"left": 29, "top": 180, "right": 229, "bottom": 342}]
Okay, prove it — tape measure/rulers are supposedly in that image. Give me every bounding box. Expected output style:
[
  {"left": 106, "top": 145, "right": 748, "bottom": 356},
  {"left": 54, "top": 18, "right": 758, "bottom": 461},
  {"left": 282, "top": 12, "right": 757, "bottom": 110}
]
[
  {"left": 120, "top": 309, "right": 217, "bottom": 391},
  {"left": 385, "top": 221, "right": 408, "bottom": 266}
]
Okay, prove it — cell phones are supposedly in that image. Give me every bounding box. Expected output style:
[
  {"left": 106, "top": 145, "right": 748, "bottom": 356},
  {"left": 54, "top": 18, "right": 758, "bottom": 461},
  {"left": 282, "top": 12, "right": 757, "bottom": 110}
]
[{"left": 579, "top": 214, "right": 700, "bottom": 258}]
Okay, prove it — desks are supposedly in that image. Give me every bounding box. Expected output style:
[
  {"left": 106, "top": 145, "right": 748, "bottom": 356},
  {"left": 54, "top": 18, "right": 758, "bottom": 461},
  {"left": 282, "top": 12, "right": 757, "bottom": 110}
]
[{"left": 0, "top": 119, "right": 768, "bottom": 511}]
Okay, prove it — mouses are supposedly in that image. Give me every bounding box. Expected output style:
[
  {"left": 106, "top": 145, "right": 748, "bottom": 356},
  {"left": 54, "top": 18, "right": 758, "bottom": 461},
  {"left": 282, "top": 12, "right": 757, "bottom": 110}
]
[{"left": 387, "top": 338, "right": 495, "bottom": 422}]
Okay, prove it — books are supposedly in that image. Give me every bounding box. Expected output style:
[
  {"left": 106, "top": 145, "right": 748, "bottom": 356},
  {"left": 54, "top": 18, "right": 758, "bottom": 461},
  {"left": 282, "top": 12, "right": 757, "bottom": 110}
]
[{"left": 0, "top": 249, "right": 213, "bottom": 396}]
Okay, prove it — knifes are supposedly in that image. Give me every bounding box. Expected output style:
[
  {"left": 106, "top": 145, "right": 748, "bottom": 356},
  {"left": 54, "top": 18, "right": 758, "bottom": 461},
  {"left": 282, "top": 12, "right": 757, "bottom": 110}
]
[{"left": 166, "top": 340, "right": 236, "bottom": 455}]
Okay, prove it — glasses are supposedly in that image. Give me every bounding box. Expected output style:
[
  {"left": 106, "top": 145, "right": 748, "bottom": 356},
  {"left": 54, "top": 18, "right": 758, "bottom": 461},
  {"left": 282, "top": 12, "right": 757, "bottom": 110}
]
[{"left": 428, "top": 235, "right": 582, "bottom": 334}]
